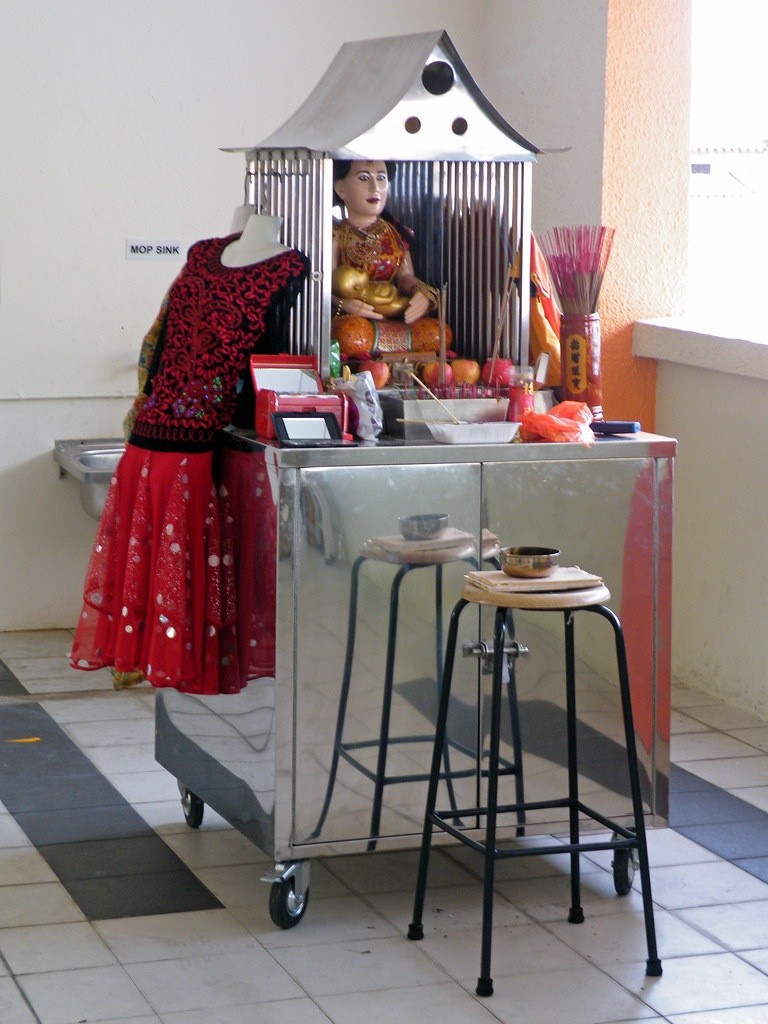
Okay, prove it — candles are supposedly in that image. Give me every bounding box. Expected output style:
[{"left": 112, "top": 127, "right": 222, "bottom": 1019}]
[{"left": 508, "top": 365, "right": 533, "bottom": 424}]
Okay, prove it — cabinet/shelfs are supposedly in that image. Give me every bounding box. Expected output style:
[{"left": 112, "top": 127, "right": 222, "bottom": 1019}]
[{"left": 155, "top": 427, "right": 679, "bottom": 930}]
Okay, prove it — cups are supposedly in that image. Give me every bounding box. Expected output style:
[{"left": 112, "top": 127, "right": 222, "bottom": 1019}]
[
  {"left": 560, "top": 312, "right": 604, "bottom": 422},
  {"left": 509, "top": 366, "right": 535, "bottom": 444}
]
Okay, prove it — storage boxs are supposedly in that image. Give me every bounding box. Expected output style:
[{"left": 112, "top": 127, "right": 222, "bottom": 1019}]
[{"left": 251, "top": 355, "right": 342, "bottom": 441}]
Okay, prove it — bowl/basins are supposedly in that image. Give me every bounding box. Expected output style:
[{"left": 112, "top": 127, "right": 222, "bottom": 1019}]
[
  {"left": 397, "top": 513, "right": 450, "bottom": 541},
  {"left": 498, "top": 546, "right": 562, "bottom": 577}
]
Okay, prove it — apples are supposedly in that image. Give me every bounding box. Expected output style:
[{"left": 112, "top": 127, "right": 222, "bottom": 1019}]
[{"left": 357, "top": 355, "right": 516, "bottom": 389}]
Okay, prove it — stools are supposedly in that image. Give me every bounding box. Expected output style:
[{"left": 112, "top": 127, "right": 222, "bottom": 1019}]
[
  {"left": 309, "top": 526, "right": 488, "bottom": 850},
  {"left": 408, "top": 589, "right": 662, "bottom": 999}
]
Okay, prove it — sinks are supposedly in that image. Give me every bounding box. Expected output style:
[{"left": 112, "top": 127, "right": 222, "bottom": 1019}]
[{"left": 51, "top": 437, "right": 130, "bottom": 525}]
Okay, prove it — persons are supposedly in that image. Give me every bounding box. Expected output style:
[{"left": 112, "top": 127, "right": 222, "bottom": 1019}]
[
  {"left": 70, "top": 211, "right": 309, "bottom": 701},
  {"left": 330, "top": 162, "right": 450, "bottom": 354}
]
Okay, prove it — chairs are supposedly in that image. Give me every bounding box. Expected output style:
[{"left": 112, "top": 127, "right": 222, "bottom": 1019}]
[{"left": 474, "top": 529, "right": 534, "bottom": 838}]
